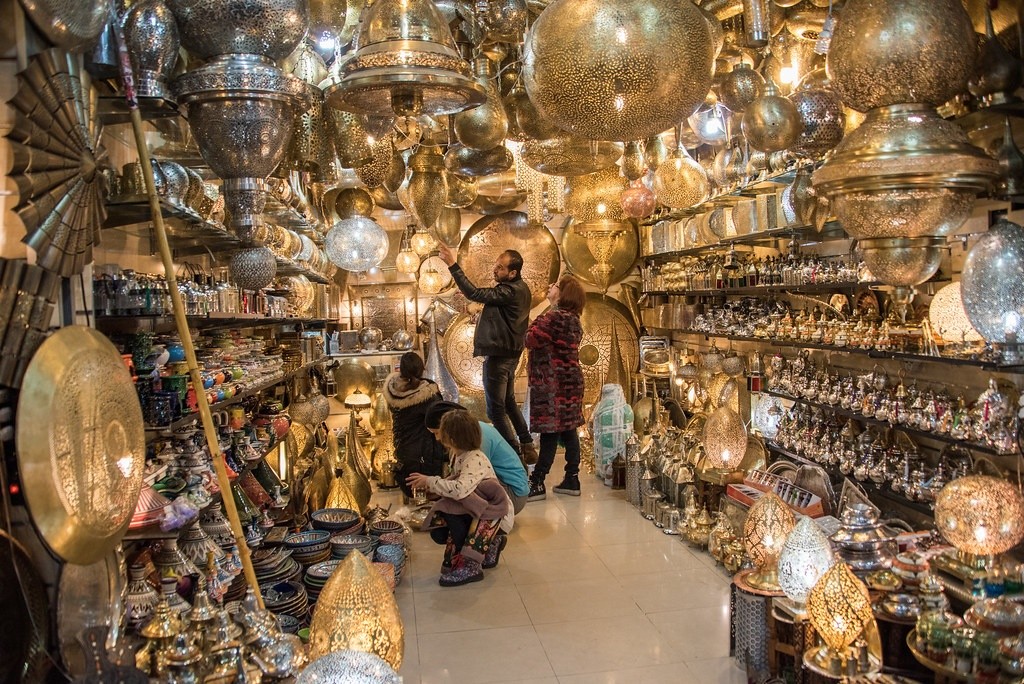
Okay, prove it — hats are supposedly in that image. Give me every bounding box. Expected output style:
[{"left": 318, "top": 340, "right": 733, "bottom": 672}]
[{"left": 424, "top": 401, "right": 468, "bottom": 429}]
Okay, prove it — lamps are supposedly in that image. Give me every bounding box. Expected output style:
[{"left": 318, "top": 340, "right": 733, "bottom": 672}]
[{"left": 0, "top": 0, "right": 1024, "bottom": 684}]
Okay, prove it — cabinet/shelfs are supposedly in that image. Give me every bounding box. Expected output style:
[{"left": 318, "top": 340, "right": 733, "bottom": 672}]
[
  {"left": 640, "top": 104, "right": 1024, "bottom": 517},
  {"left": 89, "top": 93, "right": 340, "bottom": 543}
]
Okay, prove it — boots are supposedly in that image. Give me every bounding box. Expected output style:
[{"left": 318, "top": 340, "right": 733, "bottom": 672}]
[
  {"left": 439, "top": 546, "right": 485, "bottom": 587},
  {"left": 481, "top": 535, "right": 507, "bottom": 568}
]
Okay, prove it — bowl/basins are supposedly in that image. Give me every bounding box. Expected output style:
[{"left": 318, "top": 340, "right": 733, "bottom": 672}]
[{"left": 285, "top": 509, "right": 408, "bottom": 631}]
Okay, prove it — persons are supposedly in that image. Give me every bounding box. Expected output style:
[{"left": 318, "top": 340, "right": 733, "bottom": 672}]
[
  {"left": 525, "top": 274, "right": 586, "bottom": 502},
  {"left": 383, "top": 352, "right": 442, "bottom": 504},
  {"left": 425, "top": 401, "right": 531, "bottom": 515},
  {"left": 437, "top": 239, "right": 538, "bottom": 475},
  {"left": 405, "top": 409, "right": 514, "bottom": 586}
]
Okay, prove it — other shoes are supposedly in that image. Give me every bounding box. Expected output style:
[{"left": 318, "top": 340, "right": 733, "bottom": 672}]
[{"left": 519, "top": 442, "right": 539, "bottom": 465}]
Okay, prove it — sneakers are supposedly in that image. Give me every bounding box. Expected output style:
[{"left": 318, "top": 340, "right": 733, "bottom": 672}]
[
  {"left": 526, "top": 475, "right": 546, "bottom": 501},
  {"left": 552, "top": 473, "right": 581, "bottom": 495}
]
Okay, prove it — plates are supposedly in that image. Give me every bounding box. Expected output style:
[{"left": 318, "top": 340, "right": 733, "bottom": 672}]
[{"left": 225, "top": 548, "right": 309, "bottom": 637}]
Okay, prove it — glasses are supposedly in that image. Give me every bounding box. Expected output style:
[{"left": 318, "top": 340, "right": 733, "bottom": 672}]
[{"left": 550, "top": 282, "right": 560, "bottom": 289}]
